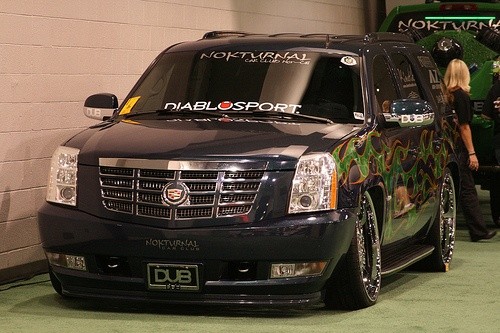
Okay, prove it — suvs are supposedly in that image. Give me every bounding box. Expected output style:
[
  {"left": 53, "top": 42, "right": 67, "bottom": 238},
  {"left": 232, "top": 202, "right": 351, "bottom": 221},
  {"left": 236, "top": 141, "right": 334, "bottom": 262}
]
[{"left": 38, "top": 31, "right": 460, "bottom": 308}]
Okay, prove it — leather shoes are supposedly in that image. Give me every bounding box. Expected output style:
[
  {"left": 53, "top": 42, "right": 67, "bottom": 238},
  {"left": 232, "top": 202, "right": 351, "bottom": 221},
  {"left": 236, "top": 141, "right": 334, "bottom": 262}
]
[{"left": 471, "top": 231, "right": 496, "bottom": 242}]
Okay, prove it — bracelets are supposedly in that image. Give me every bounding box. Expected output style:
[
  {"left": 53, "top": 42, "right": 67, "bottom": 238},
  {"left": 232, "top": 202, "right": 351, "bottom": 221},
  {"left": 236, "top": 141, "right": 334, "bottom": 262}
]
[{"left": 469, "top": 151, "right": 475, "bottom": 156}]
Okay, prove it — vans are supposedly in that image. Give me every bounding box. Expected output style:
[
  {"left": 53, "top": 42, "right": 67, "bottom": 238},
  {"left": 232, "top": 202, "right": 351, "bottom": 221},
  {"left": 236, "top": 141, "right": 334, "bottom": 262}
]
[{"left": 377, "top": 2, "right": 500, "bottom": 227}]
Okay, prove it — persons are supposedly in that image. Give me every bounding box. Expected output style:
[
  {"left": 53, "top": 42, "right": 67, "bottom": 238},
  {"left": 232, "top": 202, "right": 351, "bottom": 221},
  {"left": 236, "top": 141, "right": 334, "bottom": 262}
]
[
  {"left": 481, "top": 56, "right": 500, "bottom": 226},
  {"left": 443, "top": 59, "right": 498, "bottom": 242},
  {"left": 382, "top": 99, "right": 415, "bottom": 219}
]
